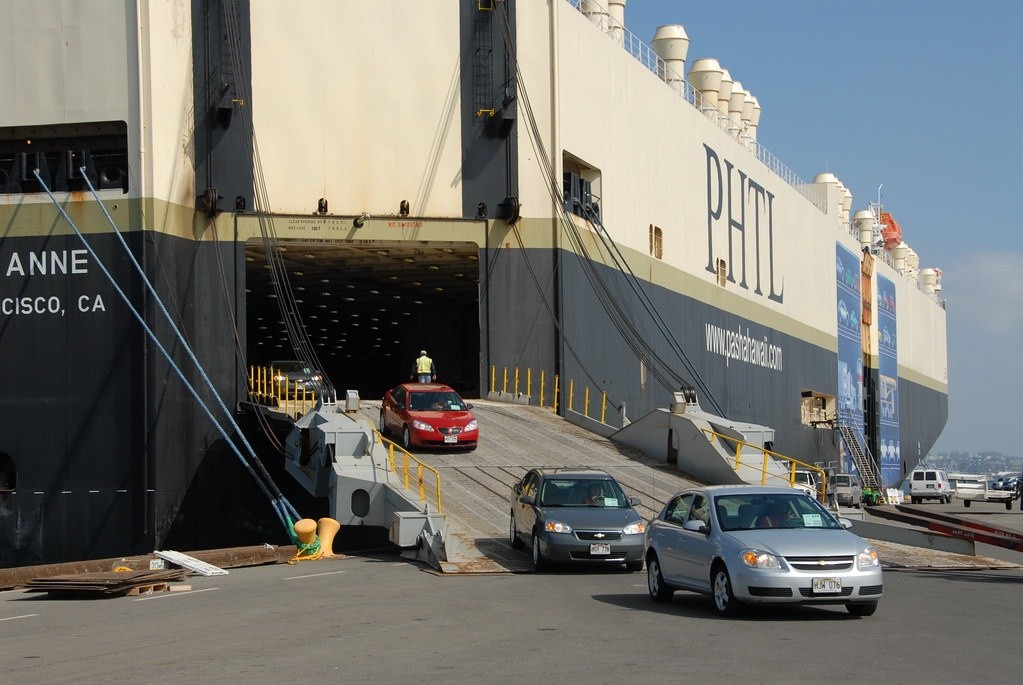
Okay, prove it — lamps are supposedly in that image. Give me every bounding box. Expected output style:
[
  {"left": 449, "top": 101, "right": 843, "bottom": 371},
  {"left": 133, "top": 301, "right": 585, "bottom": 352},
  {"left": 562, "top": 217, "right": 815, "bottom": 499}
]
[
  {"left": 317, "top": 197, "right": 329, "bottom": 215},
  {"left": 478, "top": 200, "right": 488, "bottom": 219},
  {"left": 400, "top": 199, "right": 411, "bottom": 217},
  {"left": 236, "top": 194, "right": 247, "bottom": 214}
]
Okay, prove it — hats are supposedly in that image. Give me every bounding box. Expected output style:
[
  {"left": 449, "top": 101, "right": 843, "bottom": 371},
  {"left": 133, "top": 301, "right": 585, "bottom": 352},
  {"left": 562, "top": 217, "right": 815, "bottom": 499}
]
[{"left": 420, "top": 350, "right": 427, "bottom": 354}]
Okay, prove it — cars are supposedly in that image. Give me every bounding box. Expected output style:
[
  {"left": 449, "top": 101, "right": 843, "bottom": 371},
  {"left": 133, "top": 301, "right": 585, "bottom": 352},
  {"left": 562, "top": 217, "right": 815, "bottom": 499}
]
[
  {"left": 267, "top": 360, "right": 323, "bottom": 399},
  {"left": 644, "top": 486, "right": 885, "bottom": 618},
  {"left": 992, "top": 477, "right": 1016, "bottom": 489},
  {"left": 1002, "top": 477, "right": 1023, "bottom": 492},
  {"left": 379, "top": 384, "right": 479, "bottom": 453},
  {"left": 509, "top": 467, "right": 646, "bottom": 575}
]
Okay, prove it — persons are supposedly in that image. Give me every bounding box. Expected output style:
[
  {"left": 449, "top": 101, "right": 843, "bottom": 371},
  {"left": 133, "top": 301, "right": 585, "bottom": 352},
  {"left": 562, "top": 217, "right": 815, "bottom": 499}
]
[
  {"left": 759, "top": 495, "right": 794, "bottom": 528},
  {"left": 432, "top": 393, "right": 452, "bottom": 410},
  {"left": 583, "top": 482, "right": 607, "bottom": 505},
  {"left": 410, "top": 350, "right": 436, "bottom": 383}
]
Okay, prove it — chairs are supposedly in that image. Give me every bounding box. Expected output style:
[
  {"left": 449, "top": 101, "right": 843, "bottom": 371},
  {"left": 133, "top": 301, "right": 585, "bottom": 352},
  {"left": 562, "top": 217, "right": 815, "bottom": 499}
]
[
  {"left": 718, "top": 505, "right": 727, "bottom": 525},
  {"left": 738, "top": 504, "right": 753, "bottom": 530},
  {"left": 544, "top": 483, "right": 563, "bottom": 505}
]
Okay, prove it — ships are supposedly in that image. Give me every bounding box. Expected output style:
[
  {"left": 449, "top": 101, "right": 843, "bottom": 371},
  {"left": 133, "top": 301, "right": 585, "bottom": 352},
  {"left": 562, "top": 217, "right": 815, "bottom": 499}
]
[{"left": 1, "top": 1, "right": 955, "bottom": 569}]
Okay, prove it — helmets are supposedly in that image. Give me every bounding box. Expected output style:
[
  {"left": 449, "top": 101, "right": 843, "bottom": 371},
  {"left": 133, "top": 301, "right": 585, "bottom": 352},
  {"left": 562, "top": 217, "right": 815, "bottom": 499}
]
[
  {"left": 765, "top": 498, "right": 789, "bottom": 518},
  {"left": 584, "top": 481, "right": 603, "bottom": 497}
]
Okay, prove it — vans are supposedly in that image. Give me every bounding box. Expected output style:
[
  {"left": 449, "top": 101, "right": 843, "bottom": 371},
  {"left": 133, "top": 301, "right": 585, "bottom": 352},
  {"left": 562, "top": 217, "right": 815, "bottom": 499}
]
[{"left": 908, "top": 470, "right": 952, "bottom": 505}]
